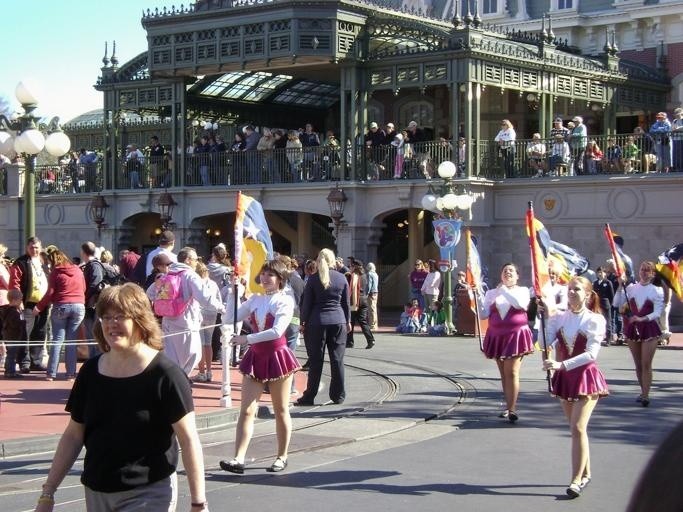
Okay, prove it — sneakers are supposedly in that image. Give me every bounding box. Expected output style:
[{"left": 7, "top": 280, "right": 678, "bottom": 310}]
[
  {"left": 67, "top": 374, "right": 76, "bottom": 381},
  {"left": 206, "top": 371, "right": 212, "bottom": 381},
  {"left": 509, "top": 410, "right": 518, "bottom": 420},
  {"left": 220, "top": 458, "right": 244, "bottom": 474},
  {"left": 501, "top": 408, "right": 509, "bottom": 417},
  {"left": 642, "top": 395, "right": 649, "bottom": 406},
  {"left": 8, "top": 373, "right": 22, "bottom": 378},
  {"left": 581, "top": 475, "right": 592, "bottom": 488},
  {"left": 637, "top": 396, "right": 642, "bottom": 401},
  {"left": 271, "top": 456, "right": 288, "bottom": 471},
  {"left": 566, "top": 481, "right": 583, "bottom": 496},
  {"left": 189, "top": 373, "right": 207, "bottom": 382}
]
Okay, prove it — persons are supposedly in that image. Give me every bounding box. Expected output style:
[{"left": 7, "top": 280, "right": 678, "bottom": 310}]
[
  {"left": 346, "top": 118, "right": 470, "bottom": 181},
  {"left": 652, "top": 280, "right": 673, "bottom": 347},
  {"left": 494, "top": 119, "right": 518, "bottom": 178},
  {"left": 99, "top": 250, "right": 118, "bottom": 278},
  {"left": 591, "top": 265, "right": 614, "bottom": 347},
  {"left": 0, "top": 244, "right": 10, "bottom": 338},
  {"left": 470, "top": 262, "right": 536, "bottom": 423},
  {"left": 146, "top": 230, "right": 177, "bottom": 279},
  {"left": 346, "top": 259, "right": 376, "bottom": 349},
  {"left": 452, "top": 271, "right": 467, "bottom": 336},
  {"left": 221, "top": 252, "right": 248, "bottom": 366},
  {"left": 671, "top": 106, "right": 683, "bottom": 172},
  {"left": 206, "top": 246, "right": 231, "bottom": 362},
  {"left": 143, "top": 254, "right": 173, "bottom": 292},
  {"left": 396, "top": 302, "right": 412, "bottom": 332},
  {"left": 366, "top": 262, "right": 379, "bottom": 329},
  {"left": 606, "top": 259, "right": 624, "bottom": 344},
  {"left": 35, "top": 283, "right": 210, "bottom": 512},
  {"left": 11, "top": 236, "right": 51, "bottom": 373},
  {"left": 421, "top": 259, "right": 442, "bottom": 312},
  {"left": 296, "top": 247, "right": 353, "bottom": 405},
  {"left": 0, "top": 123, "right": 340, "bottom": 196},
  {"left": 649, "top": 111, "right": 674, "bottom": 174},
  {"left": 219, "top": 259, "right": 303, "bottom": 474},
  {"left": 146, "top": 247, "right": 226, "bottom": 377},
  {"left": 346, "top": 256, "right": 354, "bottom": 269},
  {"left": 113, "top": 243, "right": 143, "bottom": 282},
  {"left": 535, "top": 276, "right": 610, "bottom": 496},
  {"left": 612, "top": 260, "right": 665, "bottom": 405},
  {"left": 409, "top": 258, "right": 429, "bottom": 311},
  {"left": 533, "top": 264, "right": 568, "bottom": 371},
  {"left": 32, "top": 250, "right": 87, "bottom": 381},
  {"left": 427, "top": 301, "right": 448, "bottom": 336},
  {"left": 525, "top": 114, "right": 658, "bottom": 180},
  {"left": 263, "top": 254, "right": 305, "bottom": 394},
  {"left": 335, "top": 257, "right": 350, "bottom": 275},
  {"left": 80, "top": 241, "right": 105, "bottom": 357},
  {"left": 2, "top": 287, "right": 25, "bottom": 378},
  {"left": 190, "top": 262, "right": 223, "bottom": 383},
  {"left": 407, "top": 298, "right": 422, "bottom": 333}
]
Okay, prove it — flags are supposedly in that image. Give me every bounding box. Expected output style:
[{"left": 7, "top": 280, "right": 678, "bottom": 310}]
[
  {"left": 525, "top": 208, "right": 590, "bottom": 298},
  {"left": 465, "top": 230, "right": 489, "bottom": 313},
  {"left": 233, "top": 194, "right": 274, "bottom": 298},
  {"left": 655, "top": 243, "right": 683, "bottom": 301},
  {"left": 603, "top": 226, "right": 636, "bottom": 284}
]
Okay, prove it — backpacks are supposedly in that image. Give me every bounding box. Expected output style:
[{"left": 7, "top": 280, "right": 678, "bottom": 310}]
[
  {"left": 153, "top": 269, "right": 194, "bottom": 316},
  {"left": 86, "top": 259, "right": 131, "bottom": 286}
]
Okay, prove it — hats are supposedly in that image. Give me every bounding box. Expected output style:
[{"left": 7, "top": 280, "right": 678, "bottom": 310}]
[
  {"left": 572, "top": 115, "right": 583, "bottom": 122},
  {"left": 160, "top": 230, "right": 174, "bottom": 241},
  {"left": 408, "top": 121, "right": 417, "bottom": 128},
  {"left": 552, "top": 117, "right": 562, "bottom": 123},
  {"left": 152, "top": 253, "right": 173, "bottom": 265},
  {"left": 369, "top": 122, "right": 377, "bottom": 129},
  {"left": 387, "top": 122, "right": 394, "bottom": 128}
]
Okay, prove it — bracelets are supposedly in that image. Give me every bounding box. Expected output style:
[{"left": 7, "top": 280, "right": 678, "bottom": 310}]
[{"left": 36, "top": 482, "right": 57, "bottom": 505}]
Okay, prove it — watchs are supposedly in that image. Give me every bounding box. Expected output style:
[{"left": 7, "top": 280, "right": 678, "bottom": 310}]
[{"left": 191, "top": 502, "right": 209, "bottom": 509}]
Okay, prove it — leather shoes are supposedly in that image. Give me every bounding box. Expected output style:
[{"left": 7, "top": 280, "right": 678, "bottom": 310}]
[
  {"left": 21, "top": 364, "right": 30, "bottom": 373},
  {"left": 366, "top": 340, "right": 375, "bottom": 349},
  {"left": 346, "top": 342, "right": 353, "bottom": 347},
  {"left": 30, "top": 363, "right": 47, "bottom": 371}
]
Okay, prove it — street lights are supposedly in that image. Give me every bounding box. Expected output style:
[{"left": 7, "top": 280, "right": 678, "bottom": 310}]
[
  {"left": 421, "top": 160, "right": 472, "bottom": 332},
  {"left": 193, "top": 116, "right": 219, "bottom": 136},
  {"left": 0, "top": 78, "right": 71, "bottom": 236}
]
